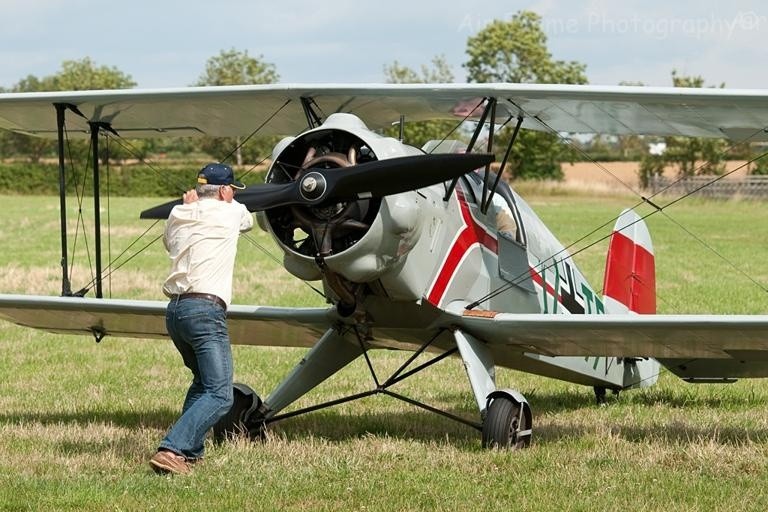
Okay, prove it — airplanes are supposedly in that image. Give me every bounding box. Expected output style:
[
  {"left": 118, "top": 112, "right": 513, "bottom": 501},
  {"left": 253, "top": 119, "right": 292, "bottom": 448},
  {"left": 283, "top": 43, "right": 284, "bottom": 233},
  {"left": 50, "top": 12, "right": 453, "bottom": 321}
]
[{"left": 0, "top": 84, "right": 767, "bottom": 451}]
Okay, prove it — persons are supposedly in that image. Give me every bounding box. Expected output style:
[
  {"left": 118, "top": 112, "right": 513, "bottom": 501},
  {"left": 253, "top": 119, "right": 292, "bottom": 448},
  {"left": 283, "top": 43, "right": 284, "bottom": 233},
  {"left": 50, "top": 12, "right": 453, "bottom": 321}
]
[{"left": 149, "top": 162, "right": 255, "bottom": 473}]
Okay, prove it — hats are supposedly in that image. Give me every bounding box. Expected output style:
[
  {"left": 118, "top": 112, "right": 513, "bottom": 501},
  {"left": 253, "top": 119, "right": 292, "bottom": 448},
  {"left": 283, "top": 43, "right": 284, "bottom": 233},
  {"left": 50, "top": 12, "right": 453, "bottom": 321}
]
[{"left": 197, "top": 164, "right": 246, "bottom": 189}]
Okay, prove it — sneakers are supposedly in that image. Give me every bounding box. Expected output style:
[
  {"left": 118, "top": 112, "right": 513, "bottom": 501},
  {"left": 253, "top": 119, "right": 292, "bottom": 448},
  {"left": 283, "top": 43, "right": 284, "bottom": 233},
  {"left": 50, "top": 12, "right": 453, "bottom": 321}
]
[{"left": 148, "top": 450, "right": 194, "bottom": 474}]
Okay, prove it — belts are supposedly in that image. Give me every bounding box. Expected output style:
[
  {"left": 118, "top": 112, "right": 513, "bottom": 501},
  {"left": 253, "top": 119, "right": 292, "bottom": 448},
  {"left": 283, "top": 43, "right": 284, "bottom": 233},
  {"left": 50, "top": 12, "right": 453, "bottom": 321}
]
[{"left": 170, "top": 293, "right": 225, "bottom": 310}]
